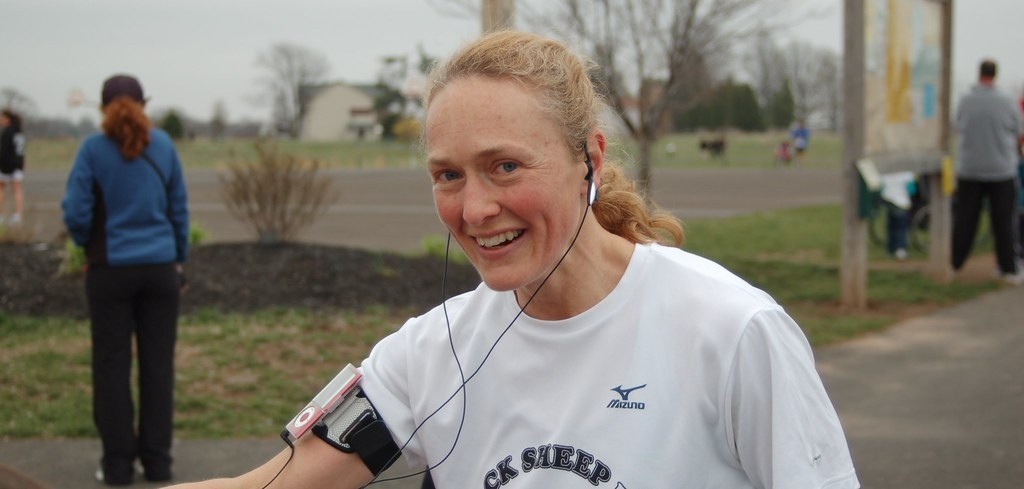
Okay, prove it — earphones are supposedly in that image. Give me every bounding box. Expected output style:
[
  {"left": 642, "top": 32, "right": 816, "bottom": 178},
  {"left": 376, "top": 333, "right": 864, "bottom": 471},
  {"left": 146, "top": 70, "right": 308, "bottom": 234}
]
[{"left": 585, "top": 160, "right": 596, "bottom": 207}]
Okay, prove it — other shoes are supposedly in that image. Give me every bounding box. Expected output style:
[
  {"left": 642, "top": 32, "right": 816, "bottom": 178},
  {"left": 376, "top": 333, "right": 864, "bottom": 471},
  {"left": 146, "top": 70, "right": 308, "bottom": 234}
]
[
  {"left": 95, "top": 470, "right": 105, "bottom": 483},
  {"left": 134, "top": 458, "right": 144, "bottom": 474}
]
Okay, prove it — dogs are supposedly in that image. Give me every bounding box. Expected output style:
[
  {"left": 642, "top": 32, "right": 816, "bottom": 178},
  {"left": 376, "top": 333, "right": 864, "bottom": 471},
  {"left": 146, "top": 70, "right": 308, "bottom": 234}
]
[{"left": 697, "top": 134, "right": 729, "bottom": 159}]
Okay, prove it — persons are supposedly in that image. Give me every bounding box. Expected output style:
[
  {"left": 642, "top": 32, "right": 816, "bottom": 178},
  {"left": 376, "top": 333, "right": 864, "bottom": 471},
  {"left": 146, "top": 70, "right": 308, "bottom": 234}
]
[
  {"left": 775, "top": 118, "right": 808, "bottom": 164},
  {"left": 950, "top": 60, "right": 1023, "bottom": 285},
  {"left": 60, "top": 73, "right": 189, "bottom": 485},
  {"left": 159, "top": 30, "right": 862, "bottom": 489},
  {"left": 0, "top": 111, "right": 27, "bottom": 222}
]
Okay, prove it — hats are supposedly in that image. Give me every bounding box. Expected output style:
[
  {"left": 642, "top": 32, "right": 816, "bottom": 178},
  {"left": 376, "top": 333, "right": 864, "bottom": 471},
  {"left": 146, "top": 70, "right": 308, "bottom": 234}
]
[{"left": 102, "top": 76, "right": 142, "bottom": 106}]
